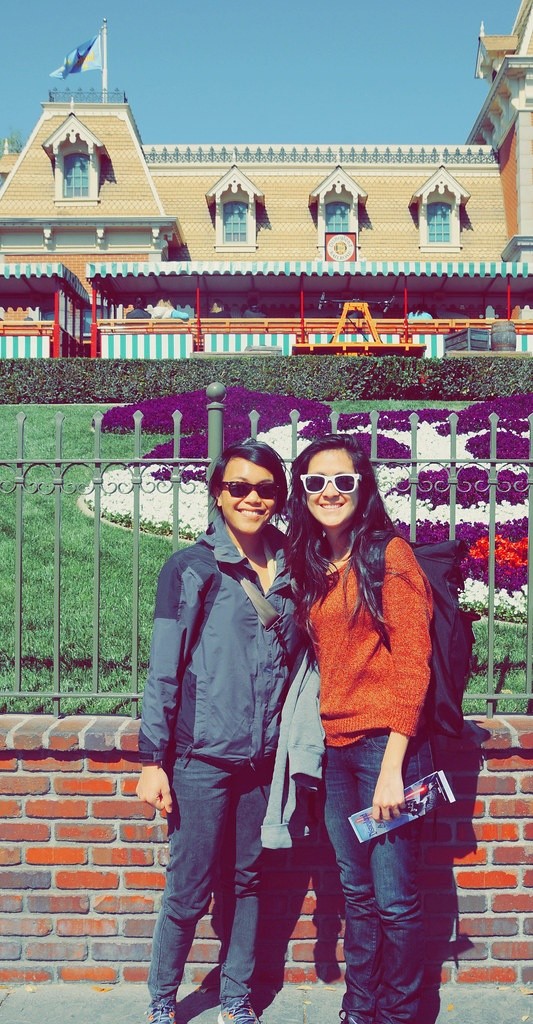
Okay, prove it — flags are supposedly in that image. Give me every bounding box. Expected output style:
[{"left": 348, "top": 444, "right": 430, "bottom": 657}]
[{"left": 48, "top": 35, "right": 102, "bottom": 78}]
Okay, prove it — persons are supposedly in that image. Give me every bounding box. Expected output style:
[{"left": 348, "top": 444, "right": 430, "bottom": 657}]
[
  {"left": 132, "top": 440, "right": 300, "bottom": 1024},
  {"left": 407, "top": 304, "right": 432, "bottom": 319},
  {"left": 209, "top": 300, "right": 231, "bottom": 318},
  {"left": 242, "top": 297, "right": 267, "bottom": 318},
  {"left": 127, "top": 296, "right": 189, "bottom": 321},
  {"left": 282, "top": 433, "right": 435, "bottom": 1024}
]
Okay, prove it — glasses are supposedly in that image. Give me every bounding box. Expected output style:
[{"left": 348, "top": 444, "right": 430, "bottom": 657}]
[
  {"left": 300, "top": 472, "right": 362, "bottom": 494},
  {"left": 221, "top": 480, "right": 281, "bottom": 499}
]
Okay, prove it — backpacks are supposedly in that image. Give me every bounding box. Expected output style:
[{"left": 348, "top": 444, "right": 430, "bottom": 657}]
[{"left": 369, "top": 528, "right": 481, "bottom": 738}]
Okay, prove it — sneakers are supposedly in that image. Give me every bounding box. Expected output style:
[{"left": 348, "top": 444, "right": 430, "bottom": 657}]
[
  {"left": 218, "top": 994, "right": 261, "bottom": 1024},
  {"left": 143, "top": 1004, "right": 176, "bottom": 1024}
]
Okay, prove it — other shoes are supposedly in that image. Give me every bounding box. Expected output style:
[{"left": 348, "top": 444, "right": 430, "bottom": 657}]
[{"left": 338, "top": 1010, "right": 366, "bottom": 1024}]
